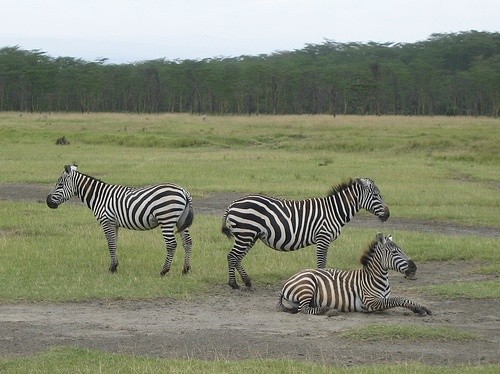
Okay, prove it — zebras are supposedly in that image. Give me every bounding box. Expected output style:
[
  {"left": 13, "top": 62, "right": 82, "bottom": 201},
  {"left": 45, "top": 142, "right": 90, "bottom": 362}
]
[
  {"left": 222, "top": 177, "right": 390, "bottom": 288},
  {"left": 46, "top": 165, "right": 193, "bottom": 275},
  {"left": 278, "top": 232, "right": 432, "bottom": 316}
]
